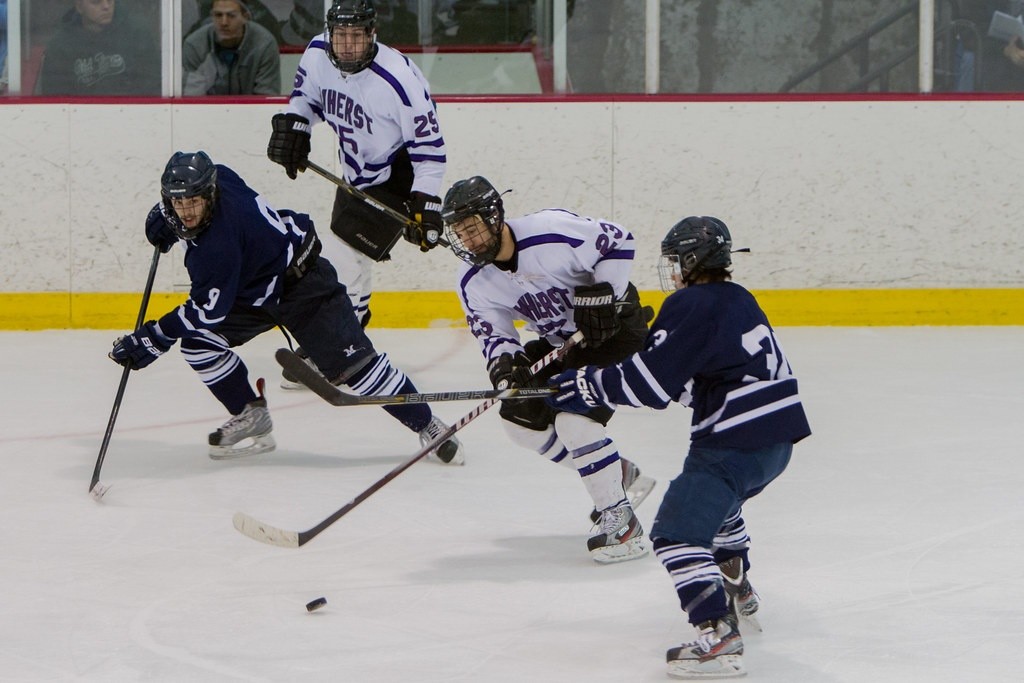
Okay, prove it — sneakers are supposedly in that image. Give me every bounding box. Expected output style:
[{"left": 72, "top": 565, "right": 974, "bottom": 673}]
[
  {"left": 418, "top": 414, "right": 466, "bottom": 467},
  {"left": 587, "top": 482, "right": 650, "bottom": 565},
  {"left": 665, "top": 603, "right": 746, "bottom": 679},
  {"left": 281, "top": 347, "right": 329, "bottom": 390},
  {"left": 718, "top": 556, "right": 762, "bottom": 632},
  {"left": 591, "top": 456, "right": 655, "bottom": 524},
  {"left": 208, "top": 377, "right": 275, "bottom": 459}
]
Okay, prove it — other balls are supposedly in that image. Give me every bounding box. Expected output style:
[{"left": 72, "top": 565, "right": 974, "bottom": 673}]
[{"left": 305, "top": 597, "right": 328, "bottom": 612}]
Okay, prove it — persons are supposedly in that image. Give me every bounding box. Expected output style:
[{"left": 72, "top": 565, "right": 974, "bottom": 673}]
[
  {"left": 267, "top": 0, "right": 446, "bottom": 390},
  {"left": 549, "top": 216, "right": 812, "bottom": 678},
  {"left": 182, "top": 0, "right": 281, "bottom": 95},
  {"left": 440, "top": 175, "right": 657, "bottom": 563},
  {"left": 30, "top": 0, "right": 162, "bottom": 97},
  {"left": 983, "top": 9, "right": 1024, "bottom": 94},
  {"left": 108, "top": 151, "right": 464, "bottom": 468}
]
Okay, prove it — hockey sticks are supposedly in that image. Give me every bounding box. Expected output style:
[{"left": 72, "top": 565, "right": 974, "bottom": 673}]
[
  {"left": 273, "top": 347, "right": 560, "bottom": 406},
  {"left": 86, "top": 245, "right": 162, "bottom": 502},
  {"left": 306, "top": 159, "right": 476, "bottom": 263},
  {"left": 232, "top": 330, "right": 584, "bottom": 548}
]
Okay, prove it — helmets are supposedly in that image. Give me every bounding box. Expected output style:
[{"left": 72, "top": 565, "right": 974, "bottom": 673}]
[
  {"left": 439, "top": 175, "right": 514, "bottom": 267},
  {"left": 159, "top": 151, "right": 218, "bottom": 241},
  {"left": 658, "top": 214, "right": 752, "bottom": 297},
  {"left": 324, "top": 0, "right": 378, "bottom": 73}
]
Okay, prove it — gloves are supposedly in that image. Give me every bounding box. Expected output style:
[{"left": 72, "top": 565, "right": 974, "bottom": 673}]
[
  {"left": 572, "top": 281, "right": 622, "bottom": 346},
  {"left": 486, "top": 350, "right": 536, "bottom": 405},
  {"left": 267, "top": 112, "right": 311, "bottom": 179},
  {"left": 108, "top": 320, "right": 177, "bottom": 370},
  {"left": 144, "top": 202, "right": 181, "bottom": 254},
  {"left": 544, "top": 364, "right": 605, "bottom": 414},
  {"left": 403, "top": 191, "right": 444, "bottom": 253}
]
